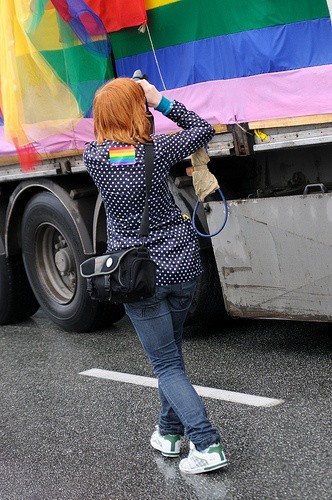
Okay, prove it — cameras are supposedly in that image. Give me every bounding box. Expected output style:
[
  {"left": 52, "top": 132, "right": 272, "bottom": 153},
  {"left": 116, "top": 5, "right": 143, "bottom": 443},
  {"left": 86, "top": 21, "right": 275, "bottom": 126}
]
[{"left": 131, "top": 69, "right": 148, "bottom": 91}]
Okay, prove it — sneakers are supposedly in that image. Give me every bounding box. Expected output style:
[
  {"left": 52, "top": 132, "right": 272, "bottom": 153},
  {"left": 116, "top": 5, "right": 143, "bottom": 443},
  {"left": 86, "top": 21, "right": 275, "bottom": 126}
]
[
  {"left": 150, "top": 425, "right": 181, "bottom": 457},
  {"left": 179, "top": 441, "right": 230, "bottom": 475}
]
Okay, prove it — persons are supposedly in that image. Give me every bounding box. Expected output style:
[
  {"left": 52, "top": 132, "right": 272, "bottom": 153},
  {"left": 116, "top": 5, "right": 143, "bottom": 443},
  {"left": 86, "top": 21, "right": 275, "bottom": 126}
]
[{"left": 83, "top": 77, "right": 230, "bottom": 475}]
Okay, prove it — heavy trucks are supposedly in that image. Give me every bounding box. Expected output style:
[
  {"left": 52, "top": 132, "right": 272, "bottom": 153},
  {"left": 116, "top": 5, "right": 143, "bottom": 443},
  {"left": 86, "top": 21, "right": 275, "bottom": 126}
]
[{"left": 0, "top": 1, "right": 332, "bottom": 334}]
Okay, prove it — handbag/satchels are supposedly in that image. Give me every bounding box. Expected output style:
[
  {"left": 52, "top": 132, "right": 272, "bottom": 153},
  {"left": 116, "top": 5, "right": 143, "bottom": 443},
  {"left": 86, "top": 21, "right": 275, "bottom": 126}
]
[{"left": 80, "top": 247, "right": 156, "bottom": 303}]
